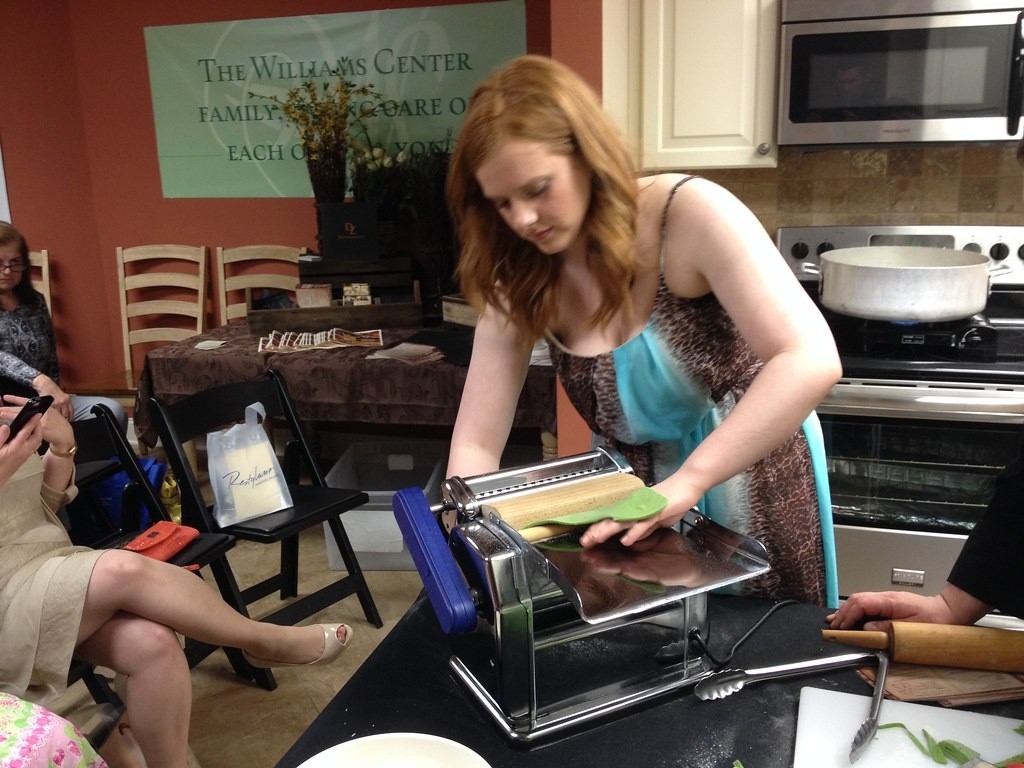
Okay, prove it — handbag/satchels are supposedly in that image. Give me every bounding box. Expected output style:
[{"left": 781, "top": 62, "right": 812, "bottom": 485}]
[
  {"left": 96, "top": 455, "right": 182, "bottom": 532},
  {"left": 124, "top": 519, "right": 199, "bottom": 563},
  {"left": 206, "top": 402, "right": 292, "bottom": 528}
]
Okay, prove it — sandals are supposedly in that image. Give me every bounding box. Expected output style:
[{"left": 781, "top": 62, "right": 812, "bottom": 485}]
[{"left": 242, "top": 623, "right": 353, "bottom": 669}]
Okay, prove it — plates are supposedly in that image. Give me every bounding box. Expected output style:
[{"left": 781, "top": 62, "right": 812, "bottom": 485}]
[
  {"left": 294, "top": 732, "right": 493, "bottom": 768},
  {"left": 792, "top": 686, "right": 1024, "bottom": 768}
]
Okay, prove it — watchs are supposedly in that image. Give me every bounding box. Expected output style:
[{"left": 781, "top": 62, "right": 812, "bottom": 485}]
[{"left": 47, "top": 441, "right": 77, "bottom": 458}]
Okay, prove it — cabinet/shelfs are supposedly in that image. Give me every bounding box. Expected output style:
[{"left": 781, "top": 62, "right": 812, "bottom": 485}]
[{"left": 600, "top": 1, "right": 780, "bottom": 171}]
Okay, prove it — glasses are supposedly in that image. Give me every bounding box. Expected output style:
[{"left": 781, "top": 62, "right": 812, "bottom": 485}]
[{"left": 0, "top": 262, "right": 29, "bottom": 272}]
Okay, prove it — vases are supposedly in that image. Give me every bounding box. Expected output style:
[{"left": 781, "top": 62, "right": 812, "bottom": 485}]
[
  {"left": 378, "top": 221, "right": 396, "bottom": 258},
  {"left": 312, "top": 201, "right": 379, "bottom": 265}
]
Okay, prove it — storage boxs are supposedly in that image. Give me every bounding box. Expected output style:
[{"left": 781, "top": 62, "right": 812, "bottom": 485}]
[{"left": 319, "top": 437, "right": 445, "bottom": 571}]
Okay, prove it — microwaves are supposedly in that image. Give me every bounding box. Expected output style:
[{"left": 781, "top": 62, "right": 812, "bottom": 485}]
[{"left": 776, "top": 0, "right": 1024, "bottom": 144}]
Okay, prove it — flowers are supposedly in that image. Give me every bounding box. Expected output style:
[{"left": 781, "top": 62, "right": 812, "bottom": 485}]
[{"left": 247, "top": 57, "right": 413, "bottom": 219}]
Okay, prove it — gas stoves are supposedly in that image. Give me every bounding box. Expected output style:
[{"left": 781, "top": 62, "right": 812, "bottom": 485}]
[{"left": 776, "top": 225, "right": 1024, "bottom": 387}]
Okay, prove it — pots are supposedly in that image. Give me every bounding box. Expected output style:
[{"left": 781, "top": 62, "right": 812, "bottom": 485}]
[{"left": 798, "top": 245, "right": 1012, "bottom": 322}]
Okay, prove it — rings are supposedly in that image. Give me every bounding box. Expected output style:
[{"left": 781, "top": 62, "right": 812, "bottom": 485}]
[{"left": 33, "top": 448, "right": 37, "bottom": 456}]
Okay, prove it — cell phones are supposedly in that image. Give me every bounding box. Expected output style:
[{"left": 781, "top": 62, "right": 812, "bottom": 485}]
[{"left": 3, "top": 396, "right": 55, "bottom": 445}]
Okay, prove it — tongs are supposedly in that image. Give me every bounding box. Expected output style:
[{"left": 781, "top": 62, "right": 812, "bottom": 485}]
[{"left": 694, "top": 649, "right": 888, "bottom": 765}]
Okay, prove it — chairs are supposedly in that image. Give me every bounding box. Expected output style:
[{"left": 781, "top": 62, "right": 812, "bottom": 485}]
[
  {"left": 25, "top": 241, "right": 302, "bottom": 485},
  {"left": 145, "top": 368, "right": 376, "bottom": 691},
  {"left": 34, "top": 403, "right": 277, "bottom": 711}
]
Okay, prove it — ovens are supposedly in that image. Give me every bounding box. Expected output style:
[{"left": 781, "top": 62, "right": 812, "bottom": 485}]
[{"left": 816, "top": 385, "right": 1024, "bottom": 631}]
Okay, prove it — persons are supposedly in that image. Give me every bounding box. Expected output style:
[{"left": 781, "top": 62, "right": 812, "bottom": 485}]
[
  {"left": 823, "top": 436, "right": 1024, "bottom": 632},
  {"left": 444, "top": 54, "right": 843, "bottom": 610},
  {"left": 0, "top": 221, "right": 75, "bottom": 423},
  {"left": 0, "top": 393, "right": 352, "bottom": 768}
]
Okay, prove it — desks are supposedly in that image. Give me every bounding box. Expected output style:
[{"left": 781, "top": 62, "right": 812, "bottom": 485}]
[{"left": 133, "top": 305, "right": 562, "bottom": 484}]
